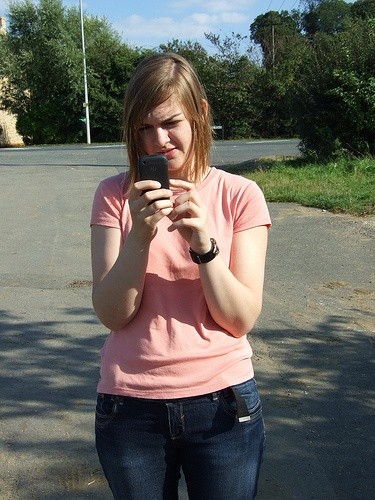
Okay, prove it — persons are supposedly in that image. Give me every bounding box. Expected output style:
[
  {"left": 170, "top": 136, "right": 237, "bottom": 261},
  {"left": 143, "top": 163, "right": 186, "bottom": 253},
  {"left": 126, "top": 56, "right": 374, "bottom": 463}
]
[{"left": 88, "top": 53, "right": 273, "bottom": 500}]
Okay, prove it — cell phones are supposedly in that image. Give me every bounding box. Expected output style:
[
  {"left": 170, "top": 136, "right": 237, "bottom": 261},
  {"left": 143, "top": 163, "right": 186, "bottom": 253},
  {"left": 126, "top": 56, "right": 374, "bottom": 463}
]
[{"left": 138, "top": 154, "right": 170, "bottom": 205}]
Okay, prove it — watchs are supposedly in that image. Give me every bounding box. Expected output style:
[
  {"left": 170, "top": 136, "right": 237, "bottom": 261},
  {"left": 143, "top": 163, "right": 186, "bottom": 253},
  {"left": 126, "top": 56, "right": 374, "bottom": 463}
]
[{"left": 188, "top": 237, "right": 220, "bottom": 266}]
